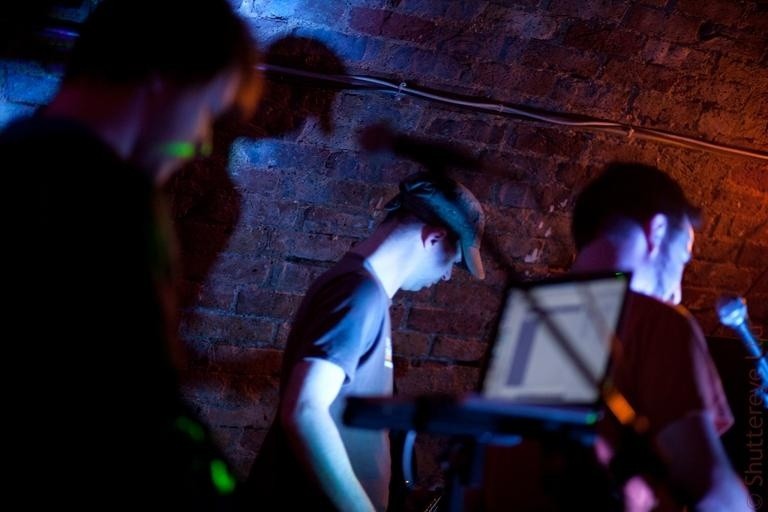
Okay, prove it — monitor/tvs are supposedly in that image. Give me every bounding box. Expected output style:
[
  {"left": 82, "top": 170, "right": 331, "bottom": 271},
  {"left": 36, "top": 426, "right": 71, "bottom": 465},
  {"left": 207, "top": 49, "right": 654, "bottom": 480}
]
[{"left": 407, "top": 271, "right": 633, "bottom": 446}]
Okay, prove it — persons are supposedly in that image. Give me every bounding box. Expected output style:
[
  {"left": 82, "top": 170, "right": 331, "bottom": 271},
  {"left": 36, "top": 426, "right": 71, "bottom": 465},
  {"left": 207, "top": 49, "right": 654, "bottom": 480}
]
[
  {"left": 0, "top": 0, "right": 222, "bottom": 512},
  {"left": 240, "top": 168, "right": 485, "bottom": 512},
  {"left": 162, "top": 35, "right": 348, "bottom": 337},
  {"left": 465, "top": 162, "right": 760, "bottom": 512}
]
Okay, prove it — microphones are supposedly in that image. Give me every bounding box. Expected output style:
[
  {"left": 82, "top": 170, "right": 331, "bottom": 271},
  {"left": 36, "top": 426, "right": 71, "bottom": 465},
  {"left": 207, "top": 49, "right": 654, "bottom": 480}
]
[{"left": 717, "top": 298, "right": 768, "bottom": 388}]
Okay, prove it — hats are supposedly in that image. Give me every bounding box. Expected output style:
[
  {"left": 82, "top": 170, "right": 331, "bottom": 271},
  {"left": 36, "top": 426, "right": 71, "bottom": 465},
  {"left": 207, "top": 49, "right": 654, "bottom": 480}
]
[{"left": 383, "top": 172, "right": 486, "bottom": 280}]
